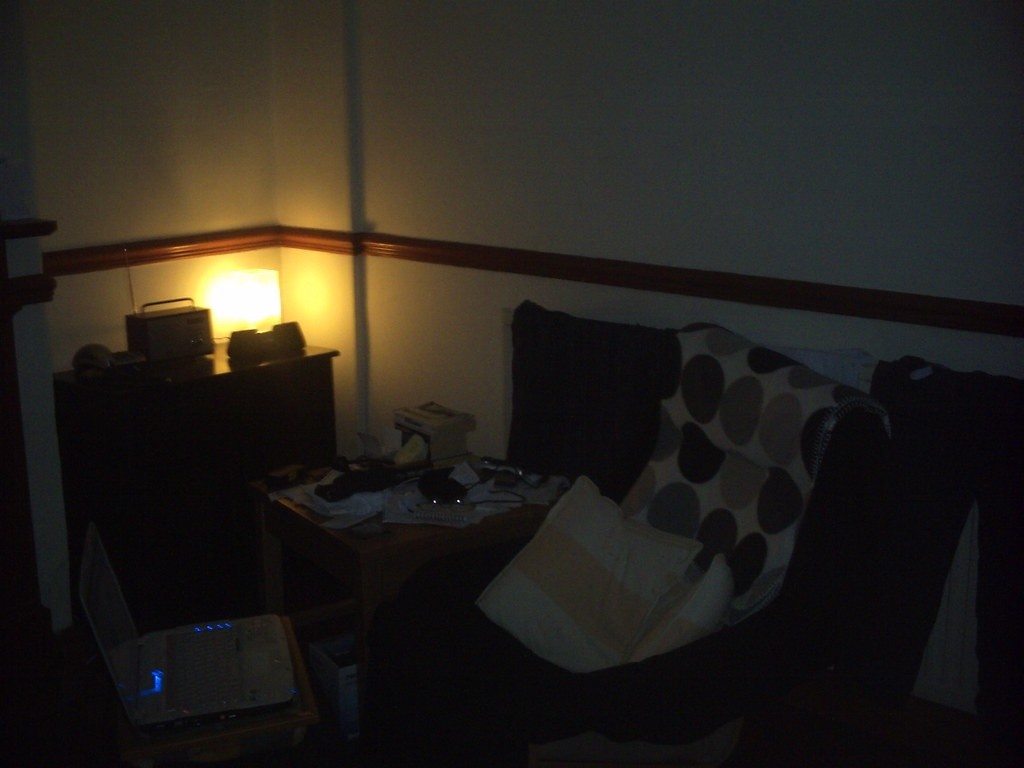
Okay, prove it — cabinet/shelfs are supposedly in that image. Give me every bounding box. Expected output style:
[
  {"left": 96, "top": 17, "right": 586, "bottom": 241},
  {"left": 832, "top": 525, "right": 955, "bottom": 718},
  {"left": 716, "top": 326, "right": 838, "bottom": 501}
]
[{"left": 52, "top": 343, "right": 339, "bottom": 632}]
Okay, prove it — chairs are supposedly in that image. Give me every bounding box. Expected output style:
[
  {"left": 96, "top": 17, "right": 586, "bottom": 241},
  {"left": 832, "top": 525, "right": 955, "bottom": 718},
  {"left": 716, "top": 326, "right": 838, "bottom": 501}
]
[{"left": 370, "top": 318, "right": 895, "bottom": 767}]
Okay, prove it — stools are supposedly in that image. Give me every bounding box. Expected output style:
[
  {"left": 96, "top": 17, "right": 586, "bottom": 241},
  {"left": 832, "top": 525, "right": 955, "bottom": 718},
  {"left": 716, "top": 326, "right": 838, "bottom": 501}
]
[{"left": 117, "top": 613, "right": 322, "bottom": 763}]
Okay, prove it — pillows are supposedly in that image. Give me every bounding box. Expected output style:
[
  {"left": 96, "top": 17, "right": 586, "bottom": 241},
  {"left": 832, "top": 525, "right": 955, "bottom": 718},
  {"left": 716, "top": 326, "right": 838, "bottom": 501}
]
[
  {"left": 474, "top": 476, "right": 703, "bottom": 674},
  {"left": 512, "top": 302, "right": 677, "bottom": 502}
]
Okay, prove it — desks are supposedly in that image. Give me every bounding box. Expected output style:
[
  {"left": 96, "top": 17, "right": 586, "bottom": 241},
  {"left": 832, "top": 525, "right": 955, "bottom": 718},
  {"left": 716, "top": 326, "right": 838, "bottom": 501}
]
[{"left": 247, "top": 457, "right": 545, "bottom": 637}]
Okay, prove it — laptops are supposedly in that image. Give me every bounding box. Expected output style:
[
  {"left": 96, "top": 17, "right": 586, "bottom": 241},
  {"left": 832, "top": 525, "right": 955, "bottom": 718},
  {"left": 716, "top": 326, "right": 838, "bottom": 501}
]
[{"left": 75, "top": 522, "right": 296, "bottom": 737}]
